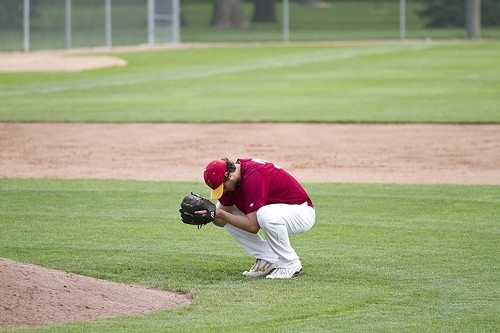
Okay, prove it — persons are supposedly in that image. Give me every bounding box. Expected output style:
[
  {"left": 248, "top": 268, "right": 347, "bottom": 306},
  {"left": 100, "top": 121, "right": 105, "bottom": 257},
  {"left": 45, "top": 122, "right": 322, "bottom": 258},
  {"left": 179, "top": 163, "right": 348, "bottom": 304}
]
[{"left": 179, "top": 158, "right": 315, "bottom": 279}]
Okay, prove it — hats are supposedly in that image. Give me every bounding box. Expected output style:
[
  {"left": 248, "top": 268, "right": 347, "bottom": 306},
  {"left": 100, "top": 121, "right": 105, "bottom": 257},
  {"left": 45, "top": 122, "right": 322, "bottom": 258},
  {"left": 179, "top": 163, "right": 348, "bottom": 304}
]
[{"left": 204, "top": 159, "right": 227, "bottom": 200}]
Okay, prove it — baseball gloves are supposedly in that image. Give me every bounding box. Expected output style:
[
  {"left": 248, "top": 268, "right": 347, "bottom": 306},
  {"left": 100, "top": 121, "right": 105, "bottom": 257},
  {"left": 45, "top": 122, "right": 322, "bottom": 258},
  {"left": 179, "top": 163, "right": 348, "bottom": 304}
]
[{"left": 179, "top": 192, "right": 216, "bottom": 229}]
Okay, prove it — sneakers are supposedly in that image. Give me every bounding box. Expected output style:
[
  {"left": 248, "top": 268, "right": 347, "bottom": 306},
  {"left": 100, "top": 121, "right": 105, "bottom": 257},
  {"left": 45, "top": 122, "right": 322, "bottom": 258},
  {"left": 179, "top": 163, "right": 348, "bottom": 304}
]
[
  {"left": 266, "top": 261, "right": 303, "bottom": 279},
  {"left": 242, "top": 258, "right": 277, "bottom": 277}
]
